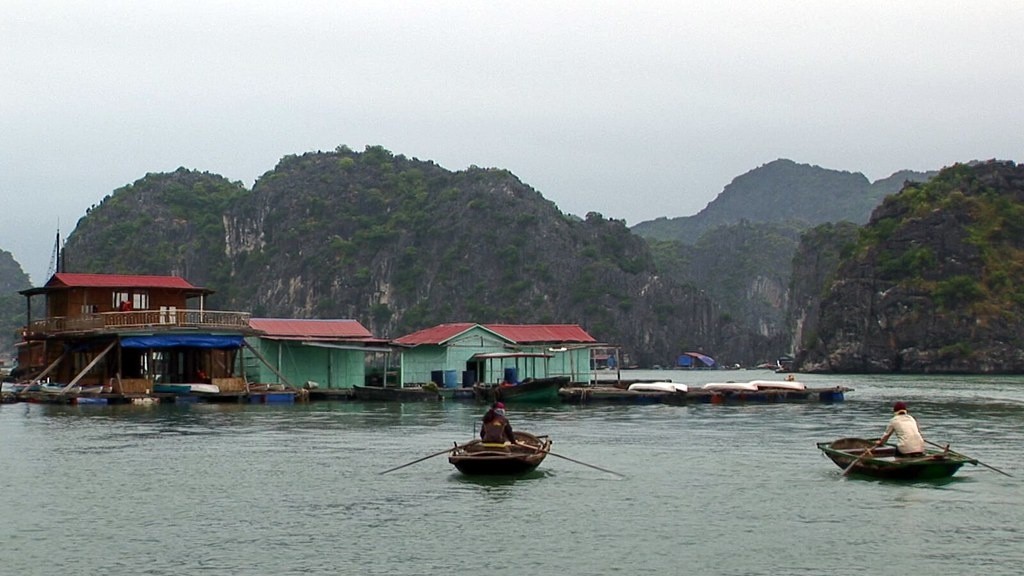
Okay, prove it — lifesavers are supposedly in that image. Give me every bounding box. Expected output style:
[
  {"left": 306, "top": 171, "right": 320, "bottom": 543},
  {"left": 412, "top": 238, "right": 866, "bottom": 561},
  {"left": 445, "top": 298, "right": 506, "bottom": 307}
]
[{"left": 123, "top": 302, "right": 133, "bottom": 311}]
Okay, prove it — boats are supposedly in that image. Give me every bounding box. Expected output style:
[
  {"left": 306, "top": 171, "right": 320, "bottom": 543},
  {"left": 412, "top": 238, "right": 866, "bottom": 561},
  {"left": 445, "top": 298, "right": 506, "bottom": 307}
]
[
  {"left": 750, "top": 380, "right": 805, "bottom": 391},
  {"left": 448, "top": 430, "right": 551, "bottom": 477},
  {"left": 701, "top": 382, "right": 759, "bottom": 392},
  {"left": 153, "top": 383, "right": 220, "bottom": 394},
  {"left": 628, "top": 382, "right": 676, "bottom": 393},
  {"left": 249, "top": 383, "right": 285, "bottom": 391},
  {"left": 0, "top": 382, "right": 113, "bottom": 394},
  {"left": 352, "top": 384, "right": 439, "bottom": 403},
  {"left": 472, "top": 353, "right": 571, "bottom": 408},
  {"left": 815, "top": 437, "right": 963, "bottom": 482},
  {"left": 654, "top": 381, "right": 688, "bottom": 393}
]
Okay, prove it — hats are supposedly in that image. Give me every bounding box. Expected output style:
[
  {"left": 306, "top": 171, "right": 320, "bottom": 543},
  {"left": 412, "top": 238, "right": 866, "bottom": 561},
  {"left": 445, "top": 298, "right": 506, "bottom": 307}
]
[
  {"left": 894, "top": 402, "right": 906, "bottom": 411},
  {"left": 493, "top": 402, "right": 504, "bottom": 409}
]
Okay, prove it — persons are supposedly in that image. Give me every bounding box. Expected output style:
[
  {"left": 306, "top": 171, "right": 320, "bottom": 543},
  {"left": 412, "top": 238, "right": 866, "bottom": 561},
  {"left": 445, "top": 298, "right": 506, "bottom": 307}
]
[
  {"left": 480, "top": 402, "right": 517, "bottom": 444},
  {"left": 875, "top": 402, "right": 924, "bottom": 457}
]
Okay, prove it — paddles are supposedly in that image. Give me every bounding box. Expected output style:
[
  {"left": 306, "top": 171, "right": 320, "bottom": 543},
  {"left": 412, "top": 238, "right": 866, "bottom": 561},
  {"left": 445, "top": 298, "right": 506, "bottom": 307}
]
[
  {"left": 378, "top": 438, "right": 482, "bottom": 475},
  {"left": 840, "top": 442, "right": 879, "bottom": 477},
  {"left": 923, "top": 437, "right": 1014, "bottom": 479},
  {"left": 516, "top": 442, "right": 626, "bottom": 479}
]
